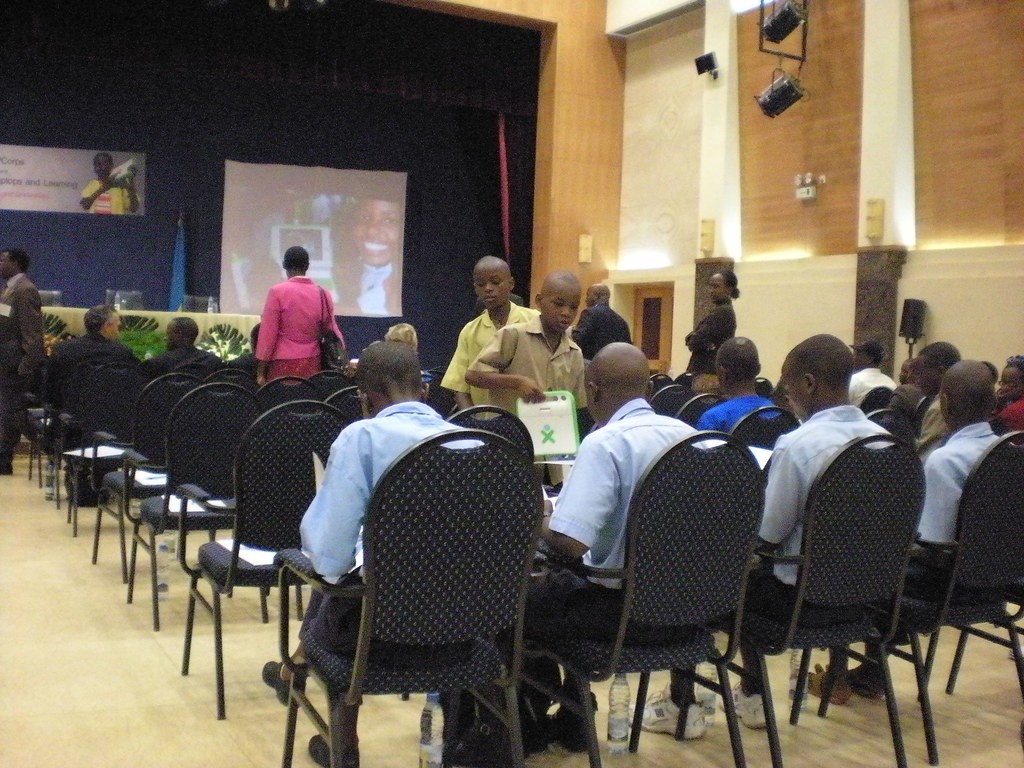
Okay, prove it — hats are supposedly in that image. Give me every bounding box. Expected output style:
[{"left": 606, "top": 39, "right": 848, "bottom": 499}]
[{"left": 849, "top": 341, "right": 885, "bottom": 363}]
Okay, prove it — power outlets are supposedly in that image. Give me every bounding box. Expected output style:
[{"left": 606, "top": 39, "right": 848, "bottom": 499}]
[{"left": 819, "top": 175, "right": 826, "bottom": 184}]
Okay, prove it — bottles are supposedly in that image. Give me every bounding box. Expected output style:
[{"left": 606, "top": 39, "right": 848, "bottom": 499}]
[
  {"left": 789, "top": 649, "right": 808, "bottom": 712},
  {"left": 606, "top": 671, "right": 631, "bottom": 755},
  {"left": 156, "top": 545, "right": 170, "bottom": 600},
  {"left": 208, "top": 297, "right": 214, "bottom": 313},
  {"left": 696, "top": 661, "right": 717, "bottom": 725},
  {"left": 418, "top": 692, "right": 443, "bottom": 768},
  {"left": 114, "top": 292, "right": 120, "bottom": 310},
  {"left": 44, "top": 465, "right": 55, "bottom": 501}
]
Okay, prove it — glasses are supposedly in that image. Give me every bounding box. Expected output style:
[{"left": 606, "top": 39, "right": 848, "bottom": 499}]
[{"left": 998, "top": 381, "right": 1017, "bottom": 387}]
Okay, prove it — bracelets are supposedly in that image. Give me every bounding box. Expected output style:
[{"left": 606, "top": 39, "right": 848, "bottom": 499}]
[{"left": 538, "top": 512, "right": 555, "bottom": 533}]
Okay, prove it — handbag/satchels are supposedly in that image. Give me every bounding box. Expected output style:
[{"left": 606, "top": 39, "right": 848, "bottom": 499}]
[
  {"left": 439, "top": 662, "right": 562, "bottom": 768},
  {"left": 318, "top": 286, "right": 342, "bottom": 376}
]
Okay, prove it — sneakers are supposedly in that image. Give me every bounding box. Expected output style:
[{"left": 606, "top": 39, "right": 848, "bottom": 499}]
[
  {"left": 848, "top": 661, "right": 884, "bottom": 699},
  {"left": 262, "top": 661, "right": 309, "bottom": 706},
  {"left": 807, "top": 664, "right": 851, "bottom": 704},
  {"left": 309, "top": 735, "right": 360, "bottom": 768},
  {"left": 715, "top": 683, "right": 766, "bottom": 728},
  {"left": 627, "top": 695, "right": 705, "bottom": 739}
]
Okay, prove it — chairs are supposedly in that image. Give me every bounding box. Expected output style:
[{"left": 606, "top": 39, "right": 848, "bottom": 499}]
[
  {"left": 675, "top": 388, "right": 723, "bottom": 419},
  {"left": 254, "top": 374, "right": 325, "bottom": 406},
  {"left": 282, "top": 428, "right": 558, "bottom": 768},
  {"left": 523, "top": 428, "right": 766, "bottom": 768},
  {"left": 422, "top": 370, "right": 456, "bottom": 417},
  {"left": 756, "top": 375, "right": 775, "bottom": 395},
  {"left": 859, "top": 386, "right": 894, "bottom": 410},
  {"left": 325, "top": 382, "right": 369, "bottom": 421},
  {"left": 666, "top": 432, "right": 925, "bottom": 768},
  {"left": 92, "top": 371, "right": 203, "bottom": 583},
  {"left": 886, "top": 426, "right": 1024, "bottom": 768},
  {"left": 17, "top": 360, "right": 69, "bottom": 483},
  {"left": 441, "top": 402, "right": 539, "bottom": 461},
  {"left": 183, "top": 294, "right": 216, "bottom": 311},
  {"left": 651, "top": 380, "right": 692, "bottom": 412},
  {"left": 300, "top": 366, "right": 351, "bottom": 399},
  {"left": 204, "top": 363, "right": 253, "bottom": 387},
  {"left": 673, "top": 371, "right": 697, "bottom": 384},
  {"left": 179, "top": 394, "right": 357, "bottom": 723},
  {"left": 649, "top": 372, "right": 676, "bottom": 391},
  {"left": 733, "top": 403, "right": 799, "bottom": 441},
  {"left": 61, "top": 361, "right": 146, "bottom": 538},
  {"left": 103, "top": 285, "right": 145, "bottom": 311},
  {"left": 37, "top": 287, "right": 65, "bottom": 305},
  {"left": 866, "top": 408, "right": 925, "bottom": 452},
  {"left": 117, "top": 374, "right": 259, "bottom": 630}
]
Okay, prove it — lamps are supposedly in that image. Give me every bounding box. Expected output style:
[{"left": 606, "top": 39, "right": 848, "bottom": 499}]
[
  {"left": 795, "top": 172, "right": 818, "bottom": 203},
  {"left": 752, "top": 0, "right": 809, "bottom": 122},
  {"left": 762, "top": 0, "right": 808, "bottom": 44}
]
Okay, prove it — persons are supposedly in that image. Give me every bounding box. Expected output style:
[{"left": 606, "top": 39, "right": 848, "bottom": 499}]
[
  {"left": 262, "top": 343, "right": 486, "bottom": 768},
  {"left": 440, "top": 257, "right": 541, "bottom": 425},
  {"left": 50, "top": 305, "right": 261, "bottom": 424},
  {"left": 336, "top": 184, "right": 404, "bottom": 318},
  {"left": 256, "top": 246, "right": 345, "bottom": 389},
  {"left": 382, "top": 322, "right": 1024, "bottom": 662},
  {"left": 79, "top": 151, "right": 141, "bottom": 216},
  {"left": 0, "top": 246, "right": 46, "bottom": 475},
  {"left": 629, "top": 333, "right": 899, "bottom": 740},
  {"left": 572, "top": 284, "right": 632, "bottom": 442},
  {"left": 792, "top": 360, "right": 1016, "bottom": 707},
  {"left": 683, "top": 270, "right": 742, "bottom": 378},
  {"left": 523, "top": 342, "right": 709, "bottom": 752},
  {"left": 465, "top": 270, "right": 588, "bottom": 490}
]
